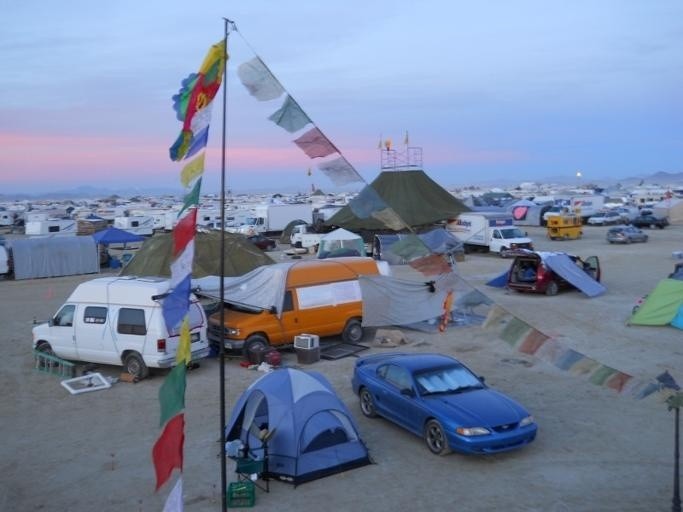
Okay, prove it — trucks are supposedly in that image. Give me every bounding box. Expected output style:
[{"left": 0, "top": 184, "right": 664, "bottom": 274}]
[{"left": 440, "top": 212, "right": 535, "bottom": 258}]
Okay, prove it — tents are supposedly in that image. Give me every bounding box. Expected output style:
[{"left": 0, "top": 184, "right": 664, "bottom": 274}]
[
  {"left": 6, "top": 233, "right": 99, "bottom": 280},
  {"left": 316, "top": 227, "right": 365, "bottom": 258},
  {"left": 93, "top": 225, "right": 144, "bottom": 268},
  {"left": 508, "top": 205, "right": 552, "bottom": 228},
  {"left": 279, "top": 218, "right": 307, "bottom": 245},
  {"left": 224, "top": 365, "right": 372, "bottom": 485},
  {"left": 653, "top": 198, "right": 683, "bottom": 223},
  {"left": 373, "top": 228, "right": 464, "bottom": 263},
  {"left": 322, "top": 170, "right": 472, "bottom": 232},
  {"left": 625, "top": 261, "right": 683, "bottom": 330},
  {"left": 119, "top": 230, "right": 276, "bottom": 278}
]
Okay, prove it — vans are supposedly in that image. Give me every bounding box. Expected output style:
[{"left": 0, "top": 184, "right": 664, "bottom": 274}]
[
  {"left": 208, "top": 254, "right": 381, "bottom": 360},
  {"left": 34, "top": 277, "right": 212, "bottom": 382},
  {"left": 505, "top": 251, "right": 600, "bottom": 297}
]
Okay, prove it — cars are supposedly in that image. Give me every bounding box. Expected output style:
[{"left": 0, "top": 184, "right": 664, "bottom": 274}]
[
  {"left": 352, "top": 355, "right": 539, "bottom": 457},
  {"left": 449, "top": 182, "right": 682, "bottom": 245}
]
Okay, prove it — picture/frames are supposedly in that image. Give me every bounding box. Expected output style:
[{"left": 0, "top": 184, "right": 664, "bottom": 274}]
[
  {"left": 60, "top": 373, "right": 111, "bottom": 395},
  {"left": 320, "top": 342, "right": 371, "bottom": 359}
]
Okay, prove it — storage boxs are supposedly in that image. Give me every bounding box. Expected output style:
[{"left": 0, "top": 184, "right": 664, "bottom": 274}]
[
  {"left": 296, "top": 349, "right": 320, "bottom": 364},
  {"left": 294, "top": 333, "right": 319, "bottom": 350}
]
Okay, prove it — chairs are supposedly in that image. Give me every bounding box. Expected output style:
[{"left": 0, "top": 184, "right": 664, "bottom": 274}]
[{"left": 234, "top": 448, "right": 269, "bottom": 493}]
